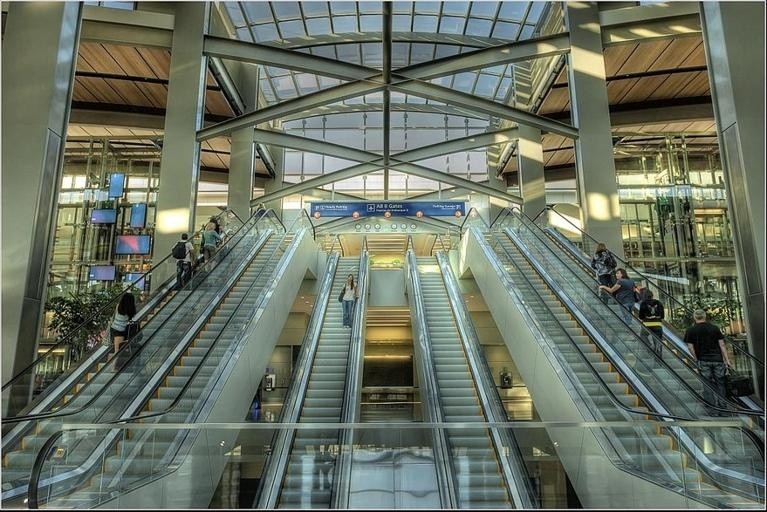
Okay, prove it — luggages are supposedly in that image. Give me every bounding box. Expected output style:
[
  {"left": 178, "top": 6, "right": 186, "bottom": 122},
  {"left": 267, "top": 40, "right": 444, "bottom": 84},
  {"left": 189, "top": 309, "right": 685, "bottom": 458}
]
[
  {"left": 220, "top": 236, "right": 230, "bottom": 261},
  {"left": 190, "top": 270, "right": 208, "bottom": 289},
  {"left": 117, "top": 320, "right": 142, "bottom": 370}
]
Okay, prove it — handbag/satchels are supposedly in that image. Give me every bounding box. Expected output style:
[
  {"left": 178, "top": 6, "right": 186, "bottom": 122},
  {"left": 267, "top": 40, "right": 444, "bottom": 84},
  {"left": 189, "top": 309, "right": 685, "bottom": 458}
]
[
  {"left": 634, "top": 291, "right": 641, "bottom": 303},
  {"left": 338, "top": 287, "right": 345, "bottom": 302},
  {"left": 609, "top": 255, "right": 617, "bottom": 267},
  {"left": 723, "top": 372, "right": 755, "bottom": 397},
  {"left": 124, "top": 323, "right": 143, "bottom": 342}
]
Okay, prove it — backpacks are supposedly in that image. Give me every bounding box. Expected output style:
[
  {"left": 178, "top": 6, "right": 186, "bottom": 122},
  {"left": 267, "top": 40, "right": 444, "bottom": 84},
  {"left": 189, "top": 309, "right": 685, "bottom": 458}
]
[{"left": 172, "top": 242, "right": 190, "bottom": 259}]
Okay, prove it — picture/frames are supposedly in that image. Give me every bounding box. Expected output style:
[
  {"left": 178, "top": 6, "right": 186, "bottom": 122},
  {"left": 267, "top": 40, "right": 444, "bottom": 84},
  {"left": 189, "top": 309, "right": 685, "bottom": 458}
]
[
  {"left": 125, "top": 272, "right": 146, "bottom": 292},
  {"left": 108, "top": 172, "right": 125, "bottom": 197},
  {"left": 114, "top": 234, "right": 151, "bottom": 255},
  {"left": 88, "top": 265, "right": 116, "bottom": 281},
  {"left": 130, "top": 203, "right": 147, "bottom": 228},
  {"left": 90, "top": 208, "right": 117, "bottom": 224}
]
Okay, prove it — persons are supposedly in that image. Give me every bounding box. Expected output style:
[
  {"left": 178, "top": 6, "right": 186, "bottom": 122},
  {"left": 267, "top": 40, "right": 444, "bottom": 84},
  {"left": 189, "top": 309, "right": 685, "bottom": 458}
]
[
  {"left": 342, "top": 274, "right": 358, "bottom": 328},
  {"left": 683, "top": 309, "right": 731, "bottom": 417},
  {"left": 254, "top": 380, "right": 263, "bottom": 409},
  {"left": 209, "top": 215, "right": 224, "bottom": 234},
  {"left": 635, "top": 287, "right": 665, "bottom": 377},
  {"left": 172, "top": 233, "right": 199, "bottom": 290},
  {"left": 110, "top": 293, "right": 137, "bottom": 373},
  {"left": 599, "top": 268, "right": 641, "bottom": 333},
  {"left": 200, "top": 222, "right": 233, "bottom": 272},
  {"left": 590, "top": 242, "right": 617, "bottom": 305}
]
